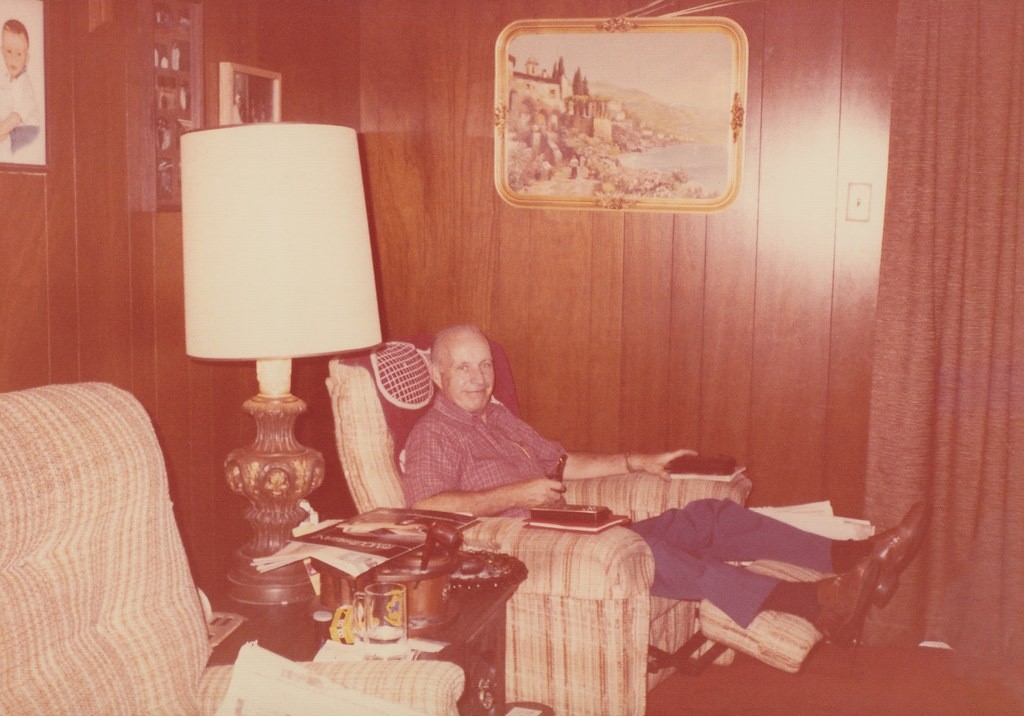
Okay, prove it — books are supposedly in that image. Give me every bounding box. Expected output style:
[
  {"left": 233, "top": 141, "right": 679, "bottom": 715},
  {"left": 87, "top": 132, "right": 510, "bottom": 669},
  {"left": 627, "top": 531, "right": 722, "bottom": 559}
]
[
  {"left": 666, "top": 466, "right": 746, "bottom": 482},
  {"left": 525, "top": 504, "right": 629, "bottom": 533}
]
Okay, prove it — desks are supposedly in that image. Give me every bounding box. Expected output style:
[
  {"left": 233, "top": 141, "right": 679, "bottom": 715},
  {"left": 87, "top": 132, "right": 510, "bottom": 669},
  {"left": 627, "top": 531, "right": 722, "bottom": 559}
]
[{"left": 206, "top": 547, "right": 525, "bottom": 716}]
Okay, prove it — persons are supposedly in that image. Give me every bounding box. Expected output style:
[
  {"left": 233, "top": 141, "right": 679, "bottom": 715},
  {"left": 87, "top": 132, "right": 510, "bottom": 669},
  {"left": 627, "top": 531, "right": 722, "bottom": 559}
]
[
  {"left": 404, "top": 326, "right": 928, "bottom": 638},
  {"left": 0, "top": 20, "right": 41, "bottom": 154}
]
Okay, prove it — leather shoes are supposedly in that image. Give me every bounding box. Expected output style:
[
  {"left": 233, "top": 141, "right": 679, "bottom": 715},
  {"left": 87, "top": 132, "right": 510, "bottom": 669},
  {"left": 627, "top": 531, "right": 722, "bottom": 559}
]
[
  {"left": 814, "top": 558, "right": 881, "bottom": 647},
  {"left": 868, "top": 501, "right": 933, "bottom": 608}
]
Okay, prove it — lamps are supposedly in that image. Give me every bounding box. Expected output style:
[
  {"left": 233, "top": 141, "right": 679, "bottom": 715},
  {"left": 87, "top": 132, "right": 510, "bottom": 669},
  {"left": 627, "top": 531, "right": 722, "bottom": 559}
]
[{"left": 180, "top": 122, "right": 383, "bottom": 604}]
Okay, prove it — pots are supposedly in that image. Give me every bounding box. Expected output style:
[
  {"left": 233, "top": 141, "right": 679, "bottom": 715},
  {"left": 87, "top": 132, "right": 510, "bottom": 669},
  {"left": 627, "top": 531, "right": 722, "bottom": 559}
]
[{"left": 370, "top": 546, "right": 455, "bottom": 617}]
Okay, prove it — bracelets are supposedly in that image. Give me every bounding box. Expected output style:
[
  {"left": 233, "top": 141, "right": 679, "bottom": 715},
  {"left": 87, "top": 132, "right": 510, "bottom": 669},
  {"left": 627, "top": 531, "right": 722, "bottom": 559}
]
[{"left": 625, "top": 451, "right": 634, "bottom": 472}]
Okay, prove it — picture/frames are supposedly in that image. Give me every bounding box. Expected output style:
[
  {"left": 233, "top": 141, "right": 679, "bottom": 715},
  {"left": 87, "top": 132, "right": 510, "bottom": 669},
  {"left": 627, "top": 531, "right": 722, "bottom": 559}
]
[
  {"left": 0, "top": 0, "right": 50, "bottom": 176},
  {"left": 219, "top": 62, "right": 283, "bottom": 125},
  {"left": 492, "top": 16, "right": 747, "bottom": 209}
]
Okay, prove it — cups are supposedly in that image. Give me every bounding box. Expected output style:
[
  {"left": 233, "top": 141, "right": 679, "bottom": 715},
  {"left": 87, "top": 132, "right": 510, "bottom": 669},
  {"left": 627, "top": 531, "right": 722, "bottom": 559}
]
[{"left": 352, "top": 583, "right": 409, "bottom": 648}]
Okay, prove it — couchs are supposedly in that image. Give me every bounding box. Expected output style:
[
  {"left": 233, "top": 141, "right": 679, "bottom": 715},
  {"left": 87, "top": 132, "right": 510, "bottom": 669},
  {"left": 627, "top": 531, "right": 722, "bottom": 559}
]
[
  {"left": 0, "top": 381, "right": 527, "bottom": 716},
  {"left": 328, "top": 342, "right": 827, "bottom": 716}
]
